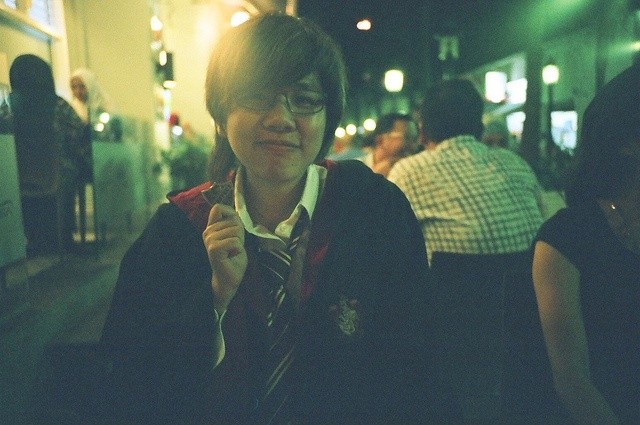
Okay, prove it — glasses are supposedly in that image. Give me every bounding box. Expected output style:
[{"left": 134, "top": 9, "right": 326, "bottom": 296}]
[{"left": 236, "top": 89, "right": 327, "bottom": 114}]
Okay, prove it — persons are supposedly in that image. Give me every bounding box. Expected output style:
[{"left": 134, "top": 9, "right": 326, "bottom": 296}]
[
  {"left": 0, "top": 53, "right": 86, "bottom": 259},
  {"left": 385, "top": 79, "right": 549, "bottom": 272},
  {"left": 351, "top": 113, "right": 419, "bottom": 175},
  {"left": 68, "top": 67, "right": 106, "bottom": 125},
  {"left": 530, "top": 63, "right": 640, "bottom": 425},
  {"left": 96, "top": 12, "right": 443, "bottom": 425}
]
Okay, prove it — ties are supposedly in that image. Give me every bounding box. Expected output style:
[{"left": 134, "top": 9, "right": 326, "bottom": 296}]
[{"left": 245, "top": 211, "right": 307, "bottom": 424}]
